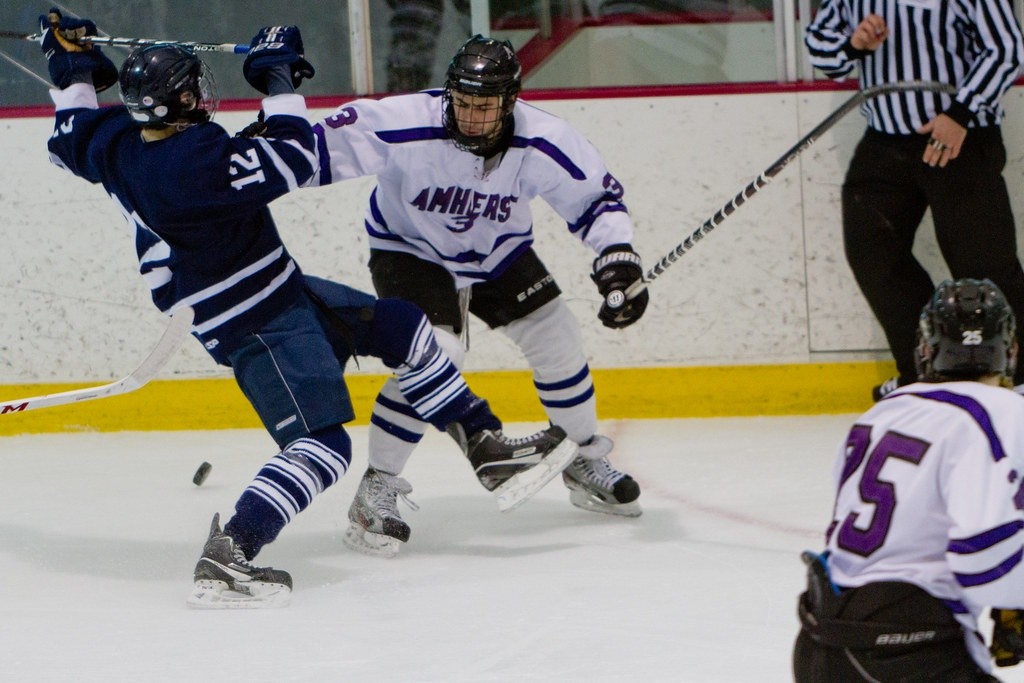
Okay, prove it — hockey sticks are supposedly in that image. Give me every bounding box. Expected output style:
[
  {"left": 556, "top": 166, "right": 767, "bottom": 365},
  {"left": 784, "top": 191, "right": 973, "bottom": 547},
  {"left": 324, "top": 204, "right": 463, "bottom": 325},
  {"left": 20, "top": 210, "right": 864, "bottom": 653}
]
[
  {"left": 0, "top": 303, "right": 195, "bottom": 418},
  {"left": 621, "top": 79, "right": 957, "bottom": 307},
  {"left": 0, "top": 29, "right": 317, "bottom": 80}
]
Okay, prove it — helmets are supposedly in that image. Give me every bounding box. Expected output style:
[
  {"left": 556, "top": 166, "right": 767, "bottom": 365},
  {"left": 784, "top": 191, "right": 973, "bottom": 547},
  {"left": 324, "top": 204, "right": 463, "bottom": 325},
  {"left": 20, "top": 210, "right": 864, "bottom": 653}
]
[
  {"left": 441, "top": 34, "right": 522, "bottom": 153},
  {"left": 912, "top": 277, "right": 1017, "bottom": 383},
  {"left": 117, "top": 43, "right": 221, "bottom": 130}
]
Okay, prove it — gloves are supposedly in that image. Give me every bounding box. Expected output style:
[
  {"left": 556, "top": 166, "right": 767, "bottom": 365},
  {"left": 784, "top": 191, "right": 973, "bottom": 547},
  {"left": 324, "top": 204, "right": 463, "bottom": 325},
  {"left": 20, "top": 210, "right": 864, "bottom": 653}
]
[
  {"left": 243, "top": 25, "right": 315, "bottom": 95},
  {"left": 589, "top": 243, "right": 649, "bottom": 330},
  {"left": 989, "top": 607, "right": 1024, "bottom": 667},
  {"left": 39, "top": 7, "right": 119, "bottom": 94},
  {"left": 235, "top": 109, "right": 268, "bottom": 139}
]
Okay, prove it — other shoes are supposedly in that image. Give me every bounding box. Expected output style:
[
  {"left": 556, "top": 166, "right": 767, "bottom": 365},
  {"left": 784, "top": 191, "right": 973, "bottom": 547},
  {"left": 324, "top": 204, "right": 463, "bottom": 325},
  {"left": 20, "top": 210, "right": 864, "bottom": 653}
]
[{"left": 872, "top": 376, "right": 901, "bottom": 402}]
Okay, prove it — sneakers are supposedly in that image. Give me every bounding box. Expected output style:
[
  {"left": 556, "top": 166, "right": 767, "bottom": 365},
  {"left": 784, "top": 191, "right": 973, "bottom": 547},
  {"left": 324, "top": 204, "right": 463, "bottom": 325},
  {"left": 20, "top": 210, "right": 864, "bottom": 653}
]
[
  {"left": 341, "top": 465, "right": 420, "bottom": 559},
  {"left": 561, "top": 434, "right": 644, "bottom": 517},
  {"left": 444, "top": 422, "right": 579, "bottom": 512},
  {"left": 186, "top": 512, "right": 293, "bottom": 611}
]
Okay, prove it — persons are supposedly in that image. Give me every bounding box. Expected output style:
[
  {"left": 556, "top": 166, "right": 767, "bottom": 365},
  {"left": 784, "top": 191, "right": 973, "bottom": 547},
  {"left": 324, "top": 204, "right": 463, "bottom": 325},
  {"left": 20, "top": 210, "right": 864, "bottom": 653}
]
[
  {"left": 804, "top": 0, "right": 1024, "bottom": 402},
  {"left": 794, "top": 280, "right": 1024, "bottom": 683},
  {"left": 41, "top": 18, "right": 650, "bottom": 602}
]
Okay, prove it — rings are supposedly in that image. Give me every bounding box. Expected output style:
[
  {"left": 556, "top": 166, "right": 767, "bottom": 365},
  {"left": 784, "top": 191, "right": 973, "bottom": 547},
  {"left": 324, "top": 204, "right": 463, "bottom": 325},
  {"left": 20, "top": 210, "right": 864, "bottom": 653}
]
[{"left": 929, "top": 138, "right": 945, "bottom": 152}]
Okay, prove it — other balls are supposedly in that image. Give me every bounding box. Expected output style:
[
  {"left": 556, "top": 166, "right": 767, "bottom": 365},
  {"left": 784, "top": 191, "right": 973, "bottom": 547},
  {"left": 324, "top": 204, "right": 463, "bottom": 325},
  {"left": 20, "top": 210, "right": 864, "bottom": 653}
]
[{"left": 192, "top": 460, "right": 212, "bottom": 486}]
